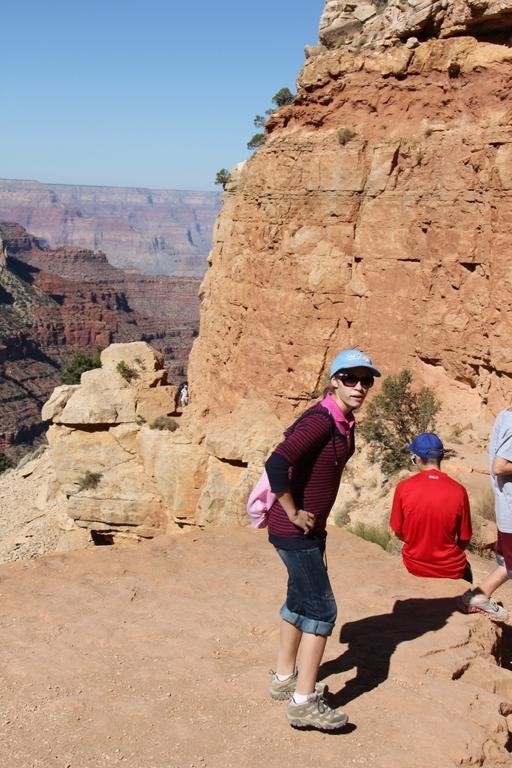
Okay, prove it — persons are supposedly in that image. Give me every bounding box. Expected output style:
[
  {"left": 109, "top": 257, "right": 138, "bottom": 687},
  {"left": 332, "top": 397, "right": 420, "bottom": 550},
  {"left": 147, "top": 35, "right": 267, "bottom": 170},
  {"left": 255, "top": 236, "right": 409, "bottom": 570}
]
[
  {"left": 179, "top": 383, "right": 188, "bottom": 407},
  {"left": 264, "top": 348, "right": 382, "bottom": 731},
  {"left": 454, "top": 401, "right": 511, "bottom": 623},
  {"left": 391, "top": 431, "right": 474, "bottom": 583}
]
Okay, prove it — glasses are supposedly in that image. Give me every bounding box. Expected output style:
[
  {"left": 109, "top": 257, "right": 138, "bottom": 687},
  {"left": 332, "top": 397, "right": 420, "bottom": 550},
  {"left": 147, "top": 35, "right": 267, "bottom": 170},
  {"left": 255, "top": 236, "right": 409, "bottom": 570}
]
[
  {"left": 335, "top": 372, "right": 375, "bottom": 389},
  {"left": 410, "top": 453, "right": 417, "bottom": 466}
]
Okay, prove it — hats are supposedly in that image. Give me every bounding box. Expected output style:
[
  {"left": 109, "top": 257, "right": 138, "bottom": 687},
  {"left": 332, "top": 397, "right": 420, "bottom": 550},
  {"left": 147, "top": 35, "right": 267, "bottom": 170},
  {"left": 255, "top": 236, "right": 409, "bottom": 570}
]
[
  {"left": 328, "top": 350, "right": 381, "bottom": 382},
  {"left": 407, "top": 433, "right": 444, "bottom": 460}
]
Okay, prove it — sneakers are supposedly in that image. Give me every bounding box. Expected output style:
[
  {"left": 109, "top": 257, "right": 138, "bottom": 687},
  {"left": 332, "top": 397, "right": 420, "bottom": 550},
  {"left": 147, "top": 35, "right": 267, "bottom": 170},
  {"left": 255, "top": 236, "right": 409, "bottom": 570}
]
[
  {"left": 457, "top": 589, "right": 510, "bottom": 622},
  {"left": 270, "top": 666, "right": 329, "bottom": 700},
  {"left": 284, "top": 691, "right": 349, "bottom": 730}
]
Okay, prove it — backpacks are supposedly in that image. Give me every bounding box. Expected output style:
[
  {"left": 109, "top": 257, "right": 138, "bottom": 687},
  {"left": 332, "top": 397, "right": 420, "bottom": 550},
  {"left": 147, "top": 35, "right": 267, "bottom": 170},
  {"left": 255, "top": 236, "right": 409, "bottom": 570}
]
[{"left": 245, "top": 407, "right": 332, "bottom": 530}]
